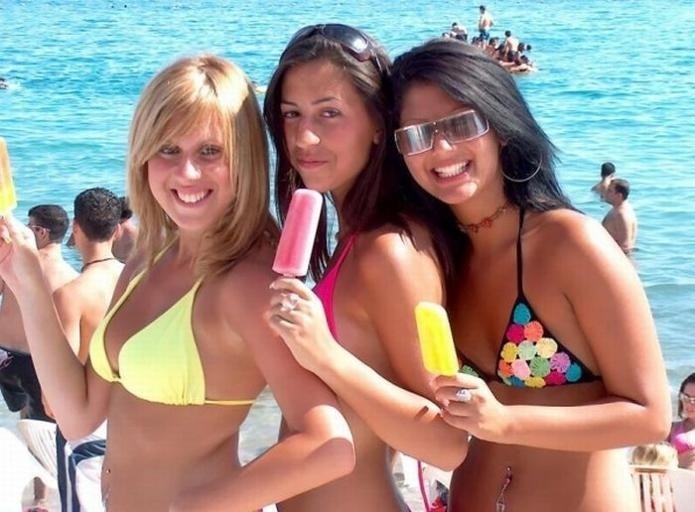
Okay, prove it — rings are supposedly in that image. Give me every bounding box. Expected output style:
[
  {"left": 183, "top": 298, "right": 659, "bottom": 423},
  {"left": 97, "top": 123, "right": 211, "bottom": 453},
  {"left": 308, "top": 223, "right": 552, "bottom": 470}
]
[
  {"left": 454, "top": 388, "right": 474, "bottom": 406},
  {"left": 287, "top": 295, "right": 301, "bottom": 311}
]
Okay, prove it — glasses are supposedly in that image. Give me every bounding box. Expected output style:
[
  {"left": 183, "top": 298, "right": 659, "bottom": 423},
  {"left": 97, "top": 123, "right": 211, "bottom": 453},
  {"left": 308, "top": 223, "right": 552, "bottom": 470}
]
[
  {"left": 392, "top": 109, "right": 492, "bottom": 156},
  {"left": 679, "top": 392, "right": 695, "bottom": 405},
  {"left": 279, "top": 23, "right": 382, "bottom": 74}
]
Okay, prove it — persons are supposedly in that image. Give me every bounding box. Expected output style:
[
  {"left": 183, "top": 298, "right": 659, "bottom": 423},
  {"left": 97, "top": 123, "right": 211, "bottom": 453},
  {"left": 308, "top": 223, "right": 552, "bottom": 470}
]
[
  {"left": 0, "top": 203, "right": 80, "bottom": 417},
  {"left": 635, "top": 443, "right": 679, "bottom": 512},
  {"left": 260, "top": 24, "right": 468, "bottom": 512},
  {"left": 602, "top": 179, "right": 638, "bottom": 254},
  {"left": 111, "top": 199, "right": 139, "bottom": 259},
  {"left": 441, "top": 4, "right": 537, "bottom": 73},
  {"left": 593, "top": 163, "right": 616, "bottom": 198},
  {"left": 40, "top": 188, "right": 128, "bottom": 512},
  {"left": 669, "top": 373, "right": 695, "bottom": 472},
  {"left": 351, "top": 38, "right": 672, "bottom": 512},
  {"left": 0, "top": 54, "right": 353, "bottom": 512}
]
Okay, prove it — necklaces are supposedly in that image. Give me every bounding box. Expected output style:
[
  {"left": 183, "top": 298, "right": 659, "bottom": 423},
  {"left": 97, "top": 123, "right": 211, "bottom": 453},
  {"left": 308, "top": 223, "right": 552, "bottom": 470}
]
[
  {"left": 80, "top": 256, "right": 117, "bottom": 272},
  {"left": 457, "top": 201, "right": 513, "bottom": 235}
]
